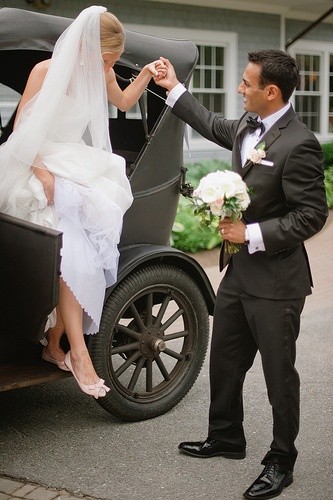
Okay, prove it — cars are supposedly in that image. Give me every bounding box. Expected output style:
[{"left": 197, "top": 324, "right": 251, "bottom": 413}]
[{"left": 0, "top": 6, "right": 219, "bottom": 424}]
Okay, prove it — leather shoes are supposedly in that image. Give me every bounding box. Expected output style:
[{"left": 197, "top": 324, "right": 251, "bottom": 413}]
[
  {"left": 243, "top": 465, "right": 292, "bottom": 500},
  {"left": 177, "top": 438, "right": 245, "bottom": 459}
]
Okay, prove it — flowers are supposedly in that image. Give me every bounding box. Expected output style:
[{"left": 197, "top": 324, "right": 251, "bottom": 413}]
[
  {"left": 193, "top": 169, "right": 250, "bottom": 254},
  {"left": 246, "top": 142, "right": 266, "bottom": 163}
]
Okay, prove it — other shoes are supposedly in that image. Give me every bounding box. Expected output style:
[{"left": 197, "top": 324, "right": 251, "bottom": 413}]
[
  {"left": 64, "top": 350, "right": 109, "bottom": 400},
  {"left": 41, "top": 350, "right": 69, "bottom": 371}
]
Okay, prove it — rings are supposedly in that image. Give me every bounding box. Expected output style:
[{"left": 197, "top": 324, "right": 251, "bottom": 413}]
[{"left": 221, "top": 228, "right": 224, "bottom": 235}]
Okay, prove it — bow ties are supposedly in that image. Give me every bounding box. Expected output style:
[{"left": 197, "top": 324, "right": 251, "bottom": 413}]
[{"left": 246, "top": 115, "right": 264, "bottom": 137}]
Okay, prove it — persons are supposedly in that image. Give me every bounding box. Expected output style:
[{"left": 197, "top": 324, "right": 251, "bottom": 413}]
[
  {"left": 0, "top": 5, "right": 168, "bottom": 398},
  {"left": 152, "top": 49, "right": 330, "bottom": 500}
]
